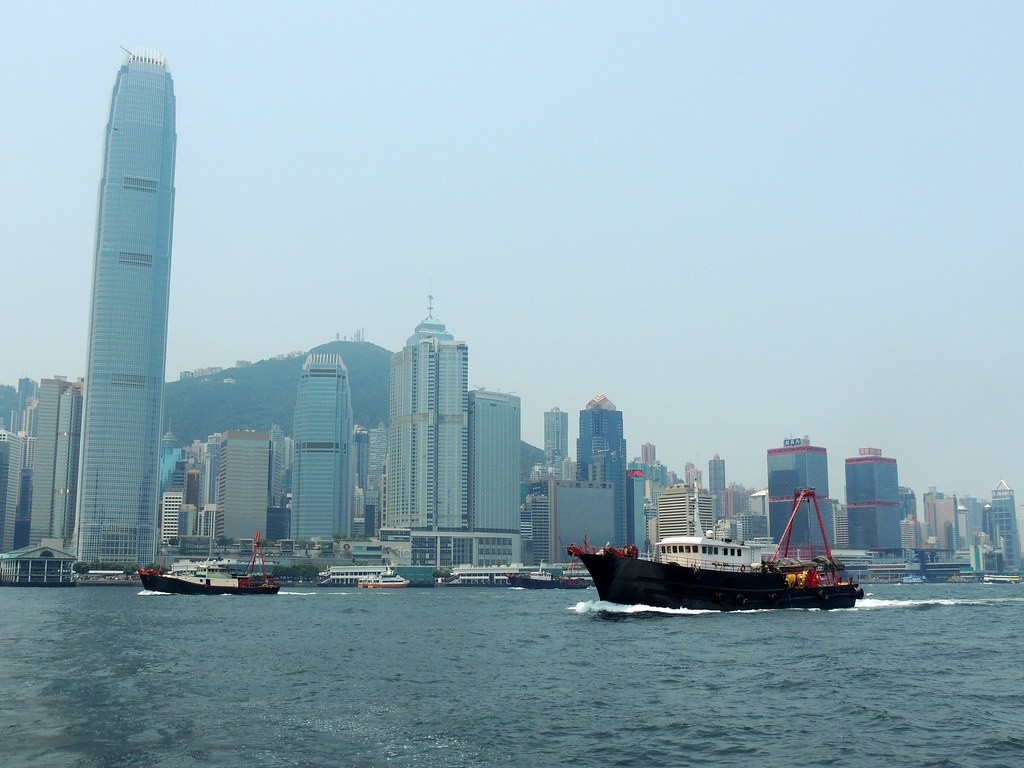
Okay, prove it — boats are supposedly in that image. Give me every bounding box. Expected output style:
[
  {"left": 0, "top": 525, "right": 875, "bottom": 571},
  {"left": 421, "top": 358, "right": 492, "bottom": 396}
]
[
  {"left": 982, "top": 573, "right": 1020, "bottom": 584},
  {"left": 899, "top": 577, "right": 924, "bottom": 584},
  {"left": 136, "top": 530, "right": 282, "bottom": 596},
  {"left": 356, "top": 562, "right": 412, "bottom": 589},
  {"left": 561, "top": 478, "right": 864, "bottom": 613},
  {"left": 504, "top": 556, "right": 593, "bottom": 589}
]
